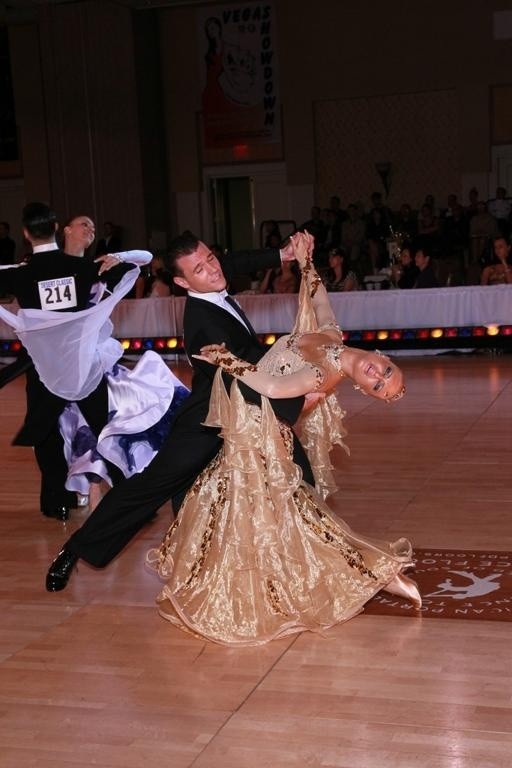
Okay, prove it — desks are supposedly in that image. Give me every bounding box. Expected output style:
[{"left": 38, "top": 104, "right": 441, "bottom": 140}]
[{"left": 0, "top": 281, "right": 511, "bottom": 355}]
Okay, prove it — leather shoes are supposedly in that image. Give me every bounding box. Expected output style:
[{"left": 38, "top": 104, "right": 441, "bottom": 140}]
[
  {"left": 54, "top": 494, "right": 89, "bottom": 520},
  {"left": 46, "top": 548, "right": 79, "bottom": 592}
]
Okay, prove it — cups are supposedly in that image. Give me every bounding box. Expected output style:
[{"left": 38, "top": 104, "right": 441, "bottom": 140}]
[{"left": 366, "top": 283, "right": 382, "bottom": 291}]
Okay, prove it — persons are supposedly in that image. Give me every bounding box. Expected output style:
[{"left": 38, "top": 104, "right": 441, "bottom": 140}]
[
  {"left": 2, "top": 222, "right": 17, "bottom": 264},
  {"left": 141, "top": 229, "right": 424, "bottom": 645},
  {"left": 304, "top": 187, "right": 511, "bottom": 293},
  {"left": 131, "top": 259, "right": 185, "bottom": 296},
  {"left": 95, "top": 222, "right": 121, "bottom": 254},
  {"left": 211, "top": 220, "right": 300, "bottom": 294},
  {"left": 45, "top": 230, "right": 316, "bottom": 592}
]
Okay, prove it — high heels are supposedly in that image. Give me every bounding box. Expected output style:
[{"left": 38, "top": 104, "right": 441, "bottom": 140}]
[{"left": 393, "top": 563, "right": 422, "bottom": 611}]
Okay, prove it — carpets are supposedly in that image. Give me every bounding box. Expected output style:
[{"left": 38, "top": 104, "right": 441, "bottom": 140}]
[{"left": 360, "top": 544, "right": 511, "bottom": 622}]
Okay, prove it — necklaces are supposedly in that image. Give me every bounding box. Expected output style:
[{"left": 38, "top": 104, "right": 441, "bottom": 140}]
[{"left": 314, "top": 340, "right": 349, "bottom": 379}]
